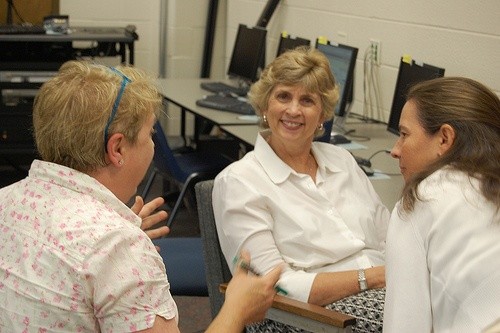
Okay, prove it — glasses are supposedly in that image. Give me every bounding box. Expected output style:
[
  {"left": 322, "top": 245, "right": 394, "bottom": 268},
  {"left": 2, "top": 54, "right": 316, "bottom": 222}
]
[{"left": 104, "top": 65, "right": 132, "bottom": 155}]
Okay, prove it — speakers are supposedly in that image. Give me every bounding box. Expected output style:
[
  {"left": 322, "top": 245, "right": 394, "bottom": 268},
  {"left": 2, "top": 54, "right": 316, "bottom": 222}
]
[{"left": 1, "top": 88, "right": 47, "bottom": 189}]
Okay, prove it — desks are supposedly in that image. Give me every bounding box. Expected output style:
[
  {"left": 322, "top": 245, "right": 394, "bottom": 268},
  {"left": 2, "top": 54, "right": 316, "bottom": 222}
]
[
  {"left": 216, "top": 121, "right": 412, "bottom": 210},
  {"left": 145, "top": 77, "right": 360, "bottom": 147}
]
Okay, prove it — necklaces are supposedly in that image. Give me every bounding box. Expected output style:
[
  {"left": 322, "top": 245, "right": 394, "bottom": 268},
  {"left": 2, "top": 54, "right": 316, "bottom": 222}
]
[{"left": 308, "top": 156, "right": 311, "bottom": 170}]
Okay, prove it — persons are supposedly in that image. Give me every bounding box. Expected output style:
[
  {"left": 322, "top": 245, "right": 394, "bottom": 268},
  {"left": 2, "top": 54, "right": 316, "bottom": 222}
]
[
  {"left": 0, "top": 62, "right": 283, "bottom": 333},
  {"left": 212, "top": 46, "right": 392, "bottom": 333},
  {"left": 383, "top": 77, "right": 500, "bottom": 333}
]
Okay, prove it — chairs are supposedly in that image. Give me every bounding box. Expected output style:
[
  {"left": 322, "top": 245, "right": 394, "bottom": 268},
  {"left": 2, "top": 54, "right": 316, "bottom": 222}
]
[{"left": 137, "top": 113, "right": 356, "bottom": 333}]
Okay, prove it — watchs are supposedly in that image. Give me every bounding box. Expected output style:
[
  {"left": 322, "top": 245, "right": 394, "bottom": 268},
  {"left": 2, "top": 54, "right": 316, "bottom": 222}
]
[{"left": 358, "top": 269, "right": 368, "bottom": 291}]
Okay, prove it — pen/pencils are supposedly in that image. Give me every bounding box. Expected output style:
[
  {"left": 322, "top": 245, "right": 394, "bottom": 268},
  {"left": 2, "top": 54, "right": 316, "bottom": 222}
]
[{"left": 233, "top": 255, "right": 288, "bottom": 296}]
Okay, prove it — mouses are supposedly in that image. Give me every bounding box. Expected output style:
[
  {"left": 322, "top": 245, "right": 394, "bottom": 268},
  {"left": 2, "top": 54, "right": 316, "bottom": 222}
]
[
  {"left": 219, "top": 91, "right": 238, "bottom": 98},
  {"left": 330, "top": 132, "right": 351, "bottom": 144},
  {"left": 353, "top": 154, "right": 372, "bottom": 169}
]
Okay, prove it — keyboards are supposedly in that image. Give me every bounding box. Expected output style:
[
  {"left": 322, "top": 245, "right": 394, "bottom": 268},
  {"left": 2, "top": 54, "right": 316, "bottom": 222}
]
[
  {"left": 201, "top": 82, "right": 245, "bottom": 97},
  {"left": 195, "top": 95, "right": 258, "bottom": 114}
]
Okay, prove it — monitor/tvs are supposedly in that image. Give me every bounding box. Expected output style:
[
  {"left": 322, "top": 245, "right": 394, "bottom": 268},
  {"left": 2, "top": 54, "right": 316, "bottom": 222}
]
[
  {"left": 226, "top": 23, "right": 267, "bottom": 82},
  {"left": 388, "top": 57, "right": 447, "bottom": 137},
  {"left": 277, "top": 32, "right": 310, "bottom": 61},
  {"left": 315, "top": 37, "right": 359, "bottom": 116}
]
